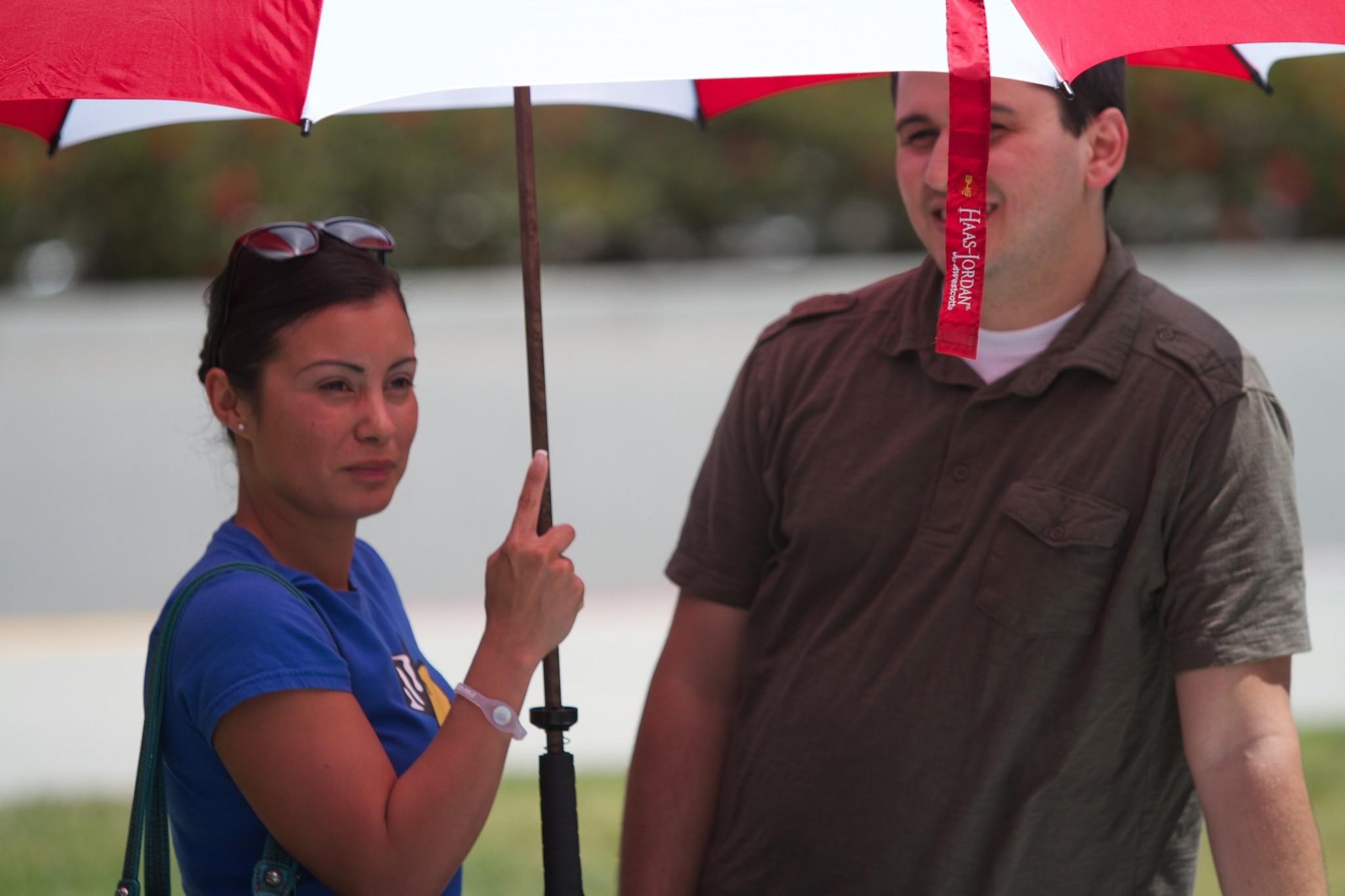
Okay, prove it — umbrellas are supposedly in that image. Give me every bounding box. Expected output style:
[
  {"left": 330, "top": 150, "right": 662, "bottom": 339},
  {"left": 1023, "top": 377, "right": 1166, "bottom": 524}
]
[{"left": 2, "top": 0, "right": 1344, "bottom": 156}]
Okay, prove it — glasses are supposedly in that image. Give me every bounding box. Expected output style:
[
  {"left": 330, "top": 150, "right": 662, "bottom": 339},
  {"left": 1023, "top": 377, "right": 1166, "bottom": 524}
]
[{"left": 212, "top": 216, "right": 395, "bottom": 368}]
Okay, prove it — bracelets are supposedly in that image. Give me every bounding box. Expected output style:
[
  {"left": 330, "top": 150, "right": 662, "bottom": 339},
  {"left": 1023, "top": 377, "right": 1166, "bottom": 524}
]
[{"left": 454, "top": 679, "right": 528, "bottom": 742}]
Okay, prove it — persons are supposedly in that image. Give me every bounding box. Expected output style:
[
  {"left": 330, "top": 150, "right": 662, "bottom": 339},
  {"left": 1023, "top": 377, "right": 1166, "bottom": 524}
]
[
  {"left": 114, "top": 215, "right": 584, "bottom": 896},
  {"left": 616, "top": 55, "right": 1327, "bottom": 896}
]
